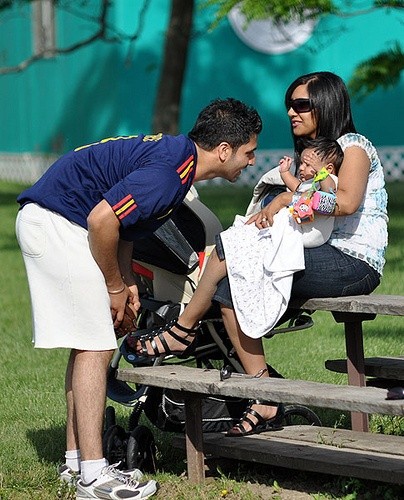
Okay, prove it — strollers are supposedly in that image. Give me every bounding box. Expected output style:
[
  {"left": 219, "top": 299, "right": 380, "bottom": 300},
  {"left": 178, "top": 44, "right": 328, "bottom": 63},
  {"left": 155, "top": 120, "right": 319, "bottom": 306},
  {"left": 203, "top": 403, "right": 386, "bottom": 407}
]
[{"left": 103, "top": 184, "right": 322, "bottom": 477}]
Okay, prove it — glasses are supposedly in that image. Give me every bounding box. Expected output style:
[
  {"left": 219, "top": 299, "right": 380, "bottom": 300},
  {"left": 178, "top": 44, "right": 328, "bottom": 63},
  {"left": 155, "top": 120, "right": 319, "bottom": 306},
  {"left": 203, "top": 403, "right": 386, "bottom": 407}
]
[{"left": 287, "top": 98, "right": 314, "bottom": 113}]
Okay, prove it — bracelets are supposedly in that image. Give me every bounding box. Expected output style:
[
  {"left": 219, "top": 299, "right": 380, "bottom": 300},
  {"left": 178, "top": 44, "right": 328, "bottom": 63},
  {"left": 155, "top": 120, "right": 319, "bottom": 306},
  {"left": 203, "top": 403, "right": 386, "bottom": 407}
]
[{"left": 107, "top": 284, "right": 125, "bottom": 294}]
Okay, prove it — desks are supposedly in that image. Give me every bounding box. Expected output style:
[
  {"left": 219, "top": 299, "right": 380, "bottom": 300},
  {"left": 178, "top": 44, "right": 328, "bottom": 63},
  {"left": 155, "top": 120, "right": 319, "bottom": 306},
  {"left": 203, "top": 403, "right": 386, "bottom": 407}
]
[{"left": 301, "top": 296, "right": 404, "bottom": 428}]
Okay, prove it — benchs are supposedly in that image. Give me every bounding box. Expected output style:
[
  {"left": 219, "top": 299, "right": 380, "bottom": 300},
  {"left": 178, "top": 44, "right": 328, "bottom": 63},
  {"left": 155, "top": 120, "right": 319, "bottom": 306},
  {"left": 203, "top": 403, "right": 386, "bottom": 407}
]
[{"left": 116, "top": 357, "right": 404, "bottom": 486}]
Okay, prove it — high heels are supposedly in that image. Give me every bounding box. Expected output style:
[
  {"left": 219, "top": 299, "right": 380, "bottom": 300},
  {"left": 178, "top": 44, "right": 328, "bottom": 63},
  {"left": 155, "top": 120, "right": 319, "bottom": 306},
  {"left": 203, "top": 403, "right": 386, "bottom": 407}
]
[
  {"left": 227, "top": 399, "right": 283, "bottom": 437},
  {"left": 127, "top": 318, "right": 202, "bottom": 359}
]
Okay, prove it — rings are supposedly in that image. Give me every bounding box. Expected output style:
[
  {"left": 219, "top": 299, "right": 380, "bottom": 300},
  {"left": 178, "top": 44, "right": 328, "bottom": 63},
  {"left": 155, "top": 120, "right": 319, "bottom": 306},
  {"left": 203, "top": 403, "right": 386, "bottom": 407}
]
[{"left": 262, "top": 218, "right": 266, "bottom": 221}]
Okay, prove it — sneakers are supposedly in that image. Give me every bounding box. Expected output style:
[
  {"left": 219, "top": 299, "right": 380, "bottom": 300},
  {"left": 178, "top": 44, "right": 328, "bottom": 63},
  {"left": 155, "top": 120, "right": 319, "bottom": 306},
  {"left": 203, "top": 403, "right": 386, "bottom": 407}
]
[
  {"left": 57, "top": 462, "right": 146, "bottom": 498},
  {"left": 76, "top": 461, "right": 158, "bottom": 500}
]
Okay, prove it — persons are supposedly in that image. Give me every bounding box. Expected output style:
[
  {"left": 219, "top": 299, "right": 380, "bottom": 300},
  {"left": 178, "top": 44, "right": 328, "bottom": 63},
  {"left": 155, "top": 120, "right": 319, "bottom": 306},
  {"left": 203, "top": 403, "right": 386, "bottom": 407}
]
[
  {"left": 16, "top": 98, "right": 262, "bottom": 500},
  {"left": 245, "top": 139, "right": 344, "bottom": 246},
  {"left": 122, "top": 70, "right": 391, "bottom": 436}
]
